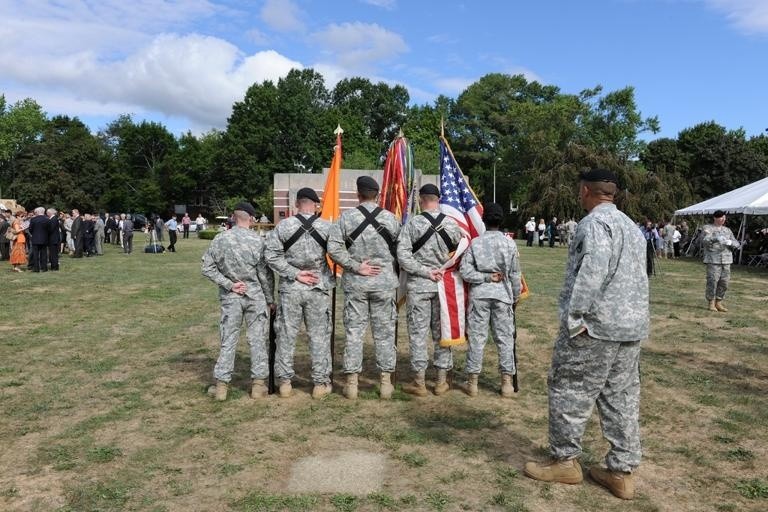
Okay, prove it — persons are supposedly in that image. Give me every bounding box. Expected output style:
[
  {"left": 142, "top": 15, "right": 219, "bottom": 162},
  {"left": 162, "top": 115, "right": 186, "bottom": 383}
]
[
  {"left": 199, "top": 201, "right": 269, "bottom": 401},
  {"left": 265, "top": 187, "right": 332, "bottom": 400},
  {"left": 325, "top": 176, "right": 401, "bottom": 399},
  {"left": 523, "top": 167, "right": 649, "bottom": 500},
  {"left": 394, "top": 183, "right": 461, "bottom": 396},
  {"left": 524, "top": 216, "right": 703, "bottom": 259},
  {"left": 0, "top": 198, "right": 205, "bottom": 271},
  {"left": 458, "top": 202, "right": 523, "bottom": 398},
  {"left": 700, "top": 209, "right": 741, "bottom": 312},
  {"left": 735, "top": 228, "right": 768, "bottom": 265}
]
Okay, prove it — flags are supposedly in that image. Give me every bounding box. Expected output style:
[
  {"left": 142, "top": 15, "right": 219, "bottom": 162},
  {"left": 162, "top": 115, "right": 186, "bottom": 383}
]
[
  {"left": 379, "top": 127, "right": 424, "bottom": 310},
  {"left": 439, "top": 135, "right": 528, "bottom": 346},
  {"left": 314, "top": 140, "right": 343, "bottom": 280}
]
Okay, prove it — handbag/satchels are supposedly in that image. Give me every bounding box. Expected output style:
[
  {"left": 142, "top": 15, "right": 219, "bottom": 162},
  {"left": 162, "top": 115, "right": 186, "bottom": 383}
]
[{"left": 4, "top": 218, "right": 18, "bottom": 241}]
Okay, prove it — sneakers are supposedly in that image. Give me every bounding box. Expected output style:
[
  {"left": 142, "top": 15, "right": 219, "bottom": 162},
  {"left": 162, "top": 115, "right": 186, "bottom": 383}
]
[{"left": 12, "top": 267, "right": 23, "bottom": 272}]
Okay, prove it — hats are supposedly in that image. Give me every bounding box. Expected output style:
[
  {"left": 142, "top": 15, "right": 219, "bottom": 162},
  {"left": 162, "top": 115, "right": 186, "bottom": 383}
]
[
  {"left": 482, "top": 202, "right": 504, "bottom": 220},
  {"left": 355, "top": 174, "right": 381, "bottom": 193},
  {"left": 418, "top": 182, "right": 442, "bottom": 198},
  {"left": 713, "top": 209, "right": 726, "bottom": 218},
  {"left": 579, "top": 166, "right": 620, "bottom": 186},
  {"left": 295, "top": 186, "right": 321, "bottom": 204},
  {"left": 232, "top": 200, "right": 256, "bottom": 217}
]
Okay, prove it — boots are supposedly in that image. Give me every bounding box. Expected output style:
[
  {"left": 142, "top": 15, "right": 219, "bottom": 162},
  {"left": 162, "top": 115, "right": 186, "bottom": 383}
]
[
  {"left": 310, "top": 382, "right": 333, "bottom": 400},
  {"left": 341, "top": 373, "right": 359, "bottom": 402},
  {"left": 206, "top": 380, "right": 230, "bottom": 402},
  {"left": 714, "top": 297, "right": 729, "bottom": 313},
  {"left": 402, "top": 372, "right": 429, "bottom": 397},
  {"left": 498, "top": 373, "right": 516, "bottom": 397},
  {"left": 273, "top": 381, "right": 295, "bottom": 400},
  {"left": 588, "top": 462, "right": 636, "bottom": 500},
  {"left": 457, "top": 373, "right": 480, "bottom": 397},
  {"left": 376, "top": 369, "right": 396, "bottom": 400},
  {"left": 249, "top": 378, "right": 271, "bottom": 399},
  {"left": 523, "top": 455, "right": 584, "bottom": 485},
  {"left": 432, "top": 369, "right": 450, "bottom": 397},
  {"left": 707, "top": 297, "right": 718, "bottom": 312}
]
